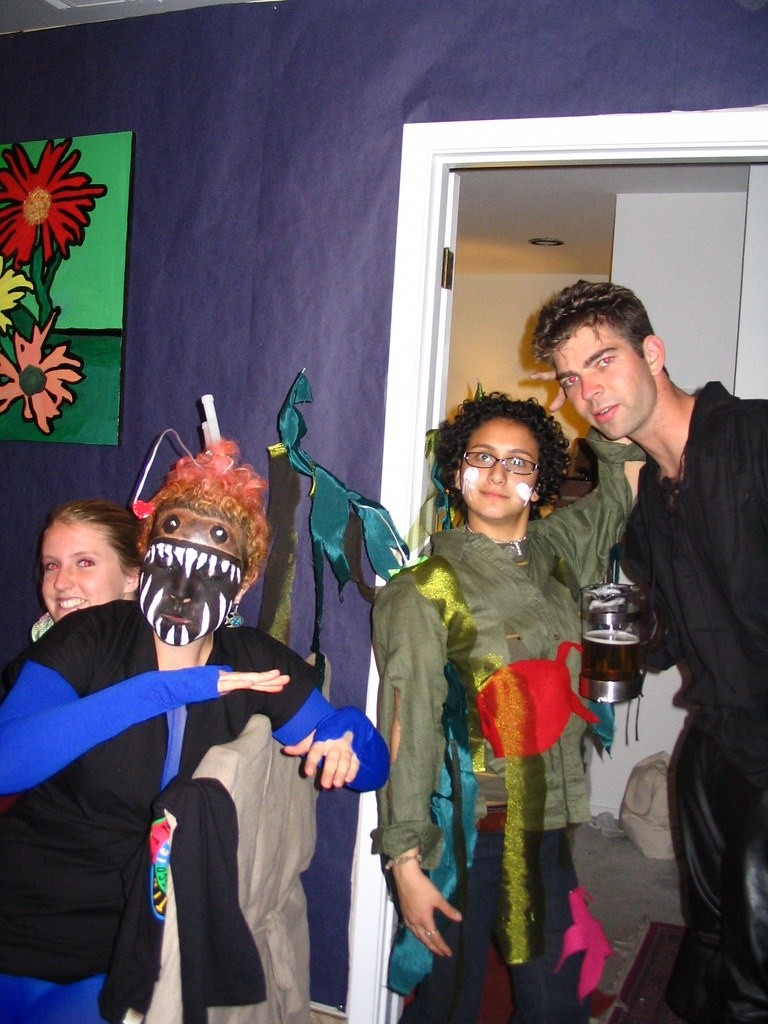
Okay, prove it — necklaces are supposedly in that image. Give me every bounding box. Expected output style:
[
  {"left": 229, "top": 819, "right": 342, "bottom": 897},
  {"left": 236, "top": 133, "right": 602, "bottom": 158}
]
[{"left": 467, "top": 526, "right": 526, "bottom": 544}]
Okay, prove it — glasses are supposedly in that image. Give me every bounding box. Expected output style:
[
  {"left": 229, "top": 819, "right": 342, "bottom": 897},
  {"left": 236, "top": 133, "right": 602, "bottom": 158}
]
[{"left": 459, "top": 451, "right": 546, "bottom": 476}]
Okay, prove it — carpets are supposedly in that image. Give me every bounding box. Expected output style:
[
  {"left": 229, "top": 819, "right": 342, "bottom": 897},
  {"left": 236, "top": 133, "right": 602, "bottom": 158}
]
[{"left": 607, "top": 920, "right": 689, "bottom": 1024}]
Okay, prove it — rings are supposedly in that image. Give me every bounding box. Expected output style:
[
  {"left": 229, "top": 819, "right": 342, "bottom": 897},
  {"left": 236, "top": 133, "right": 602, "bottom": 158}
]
[{"left": 427, "top": 930, "right": 437, "bottom": 935}]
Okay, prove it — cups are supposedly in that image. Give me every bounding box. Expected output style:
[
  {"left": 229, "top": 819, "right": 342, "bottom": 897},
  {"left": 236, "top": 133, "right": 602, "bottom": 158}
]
[{"left": 579, "top": 584, "right": 648, "bottom": 703}]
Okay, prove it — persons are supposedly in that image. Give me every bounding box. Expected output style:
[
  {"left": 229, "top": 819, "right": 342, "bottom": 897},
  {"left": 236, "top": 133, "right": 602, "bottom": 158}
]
[
  {"left": 529, "top": 280, "right": 768, "bottom": 1024},
  {"left": 32, "top": 498, "right": 141, "bottom": 642},
  {"left": 0, "top": 443, "right": 390, "bottom": 1024},
  {"left": 371, "top": 391, "right": 649, "bottom": 1024}
]
